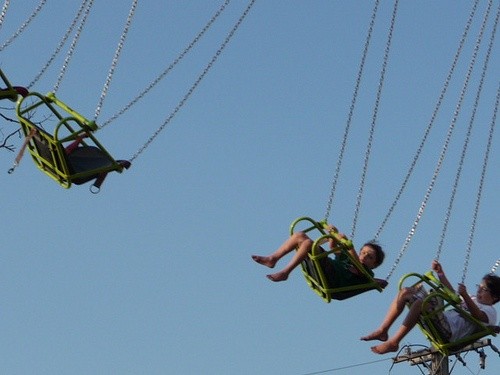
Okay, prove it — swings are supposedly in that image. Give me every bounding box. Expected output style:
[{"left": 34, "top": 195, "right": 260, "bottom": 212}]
[
  {"left": 16, "top": 0, "right": 253, "bottom": 191},
  {"left": 288, "top": 0, "right": 493, "bottom": 303},
  {"left": 397, "top": 7, "right": 499, "bottom": 362},
  {"left": 0, "top": 0, "right": 92, "bottom": 101}
]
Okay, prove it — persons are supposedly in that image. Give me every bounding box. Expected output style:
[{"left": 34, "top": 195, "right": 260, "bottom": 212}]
[
  {"left": 360, "top": 260, "right": 500, "bottom": 355},
  {"left": 251, "top": 225, "right": 385, "bottom": 288}
]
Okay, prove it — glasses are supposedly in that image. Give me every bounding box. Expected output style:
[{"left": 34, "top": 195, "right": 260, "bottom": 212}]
[{"left": 475, "top": 284, "right": 490, "bottom": 293}]
